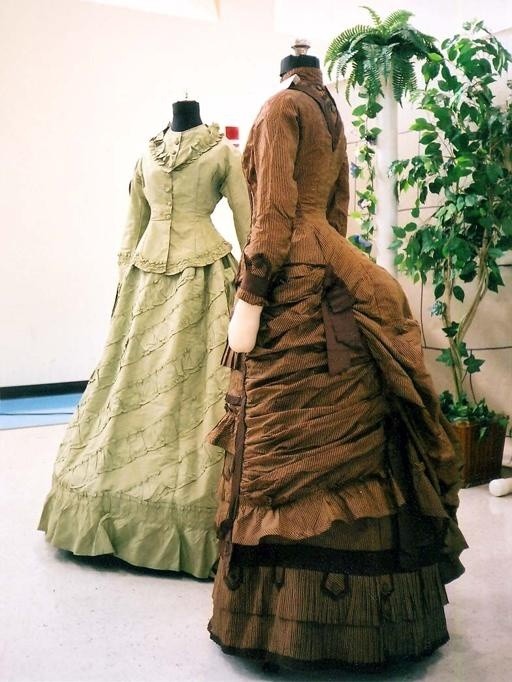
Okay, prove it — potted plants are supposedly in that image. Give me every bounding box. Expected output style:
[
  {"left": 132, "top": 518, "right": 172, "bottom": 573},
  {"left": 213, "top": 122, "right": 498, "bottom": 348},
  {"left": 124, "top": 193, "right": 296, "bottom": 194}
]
[{"left": 390, "top": 19, "right": 512, "bottom": 487}]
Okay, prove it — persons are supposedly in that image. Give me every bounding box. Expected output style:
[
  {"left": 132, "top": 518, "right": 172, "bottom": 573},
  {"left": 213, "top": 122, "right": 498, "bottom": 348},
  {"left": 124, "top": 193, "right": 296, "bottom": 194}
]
[
  {"left": 38, "top": 97, "right": 252, "bottom": 584},
  {"left": 204, "top": 49, "right": 473, "bottom": 669}
]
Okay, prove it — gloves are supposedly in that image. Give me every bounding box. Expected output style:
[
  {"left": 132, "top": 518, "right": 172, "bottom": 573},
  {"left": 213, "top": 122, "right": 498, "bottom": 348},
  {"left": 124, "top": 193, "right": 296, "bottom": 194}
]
[{"left": 228, "top": 298, "right": 264, "bottom": 353}]
